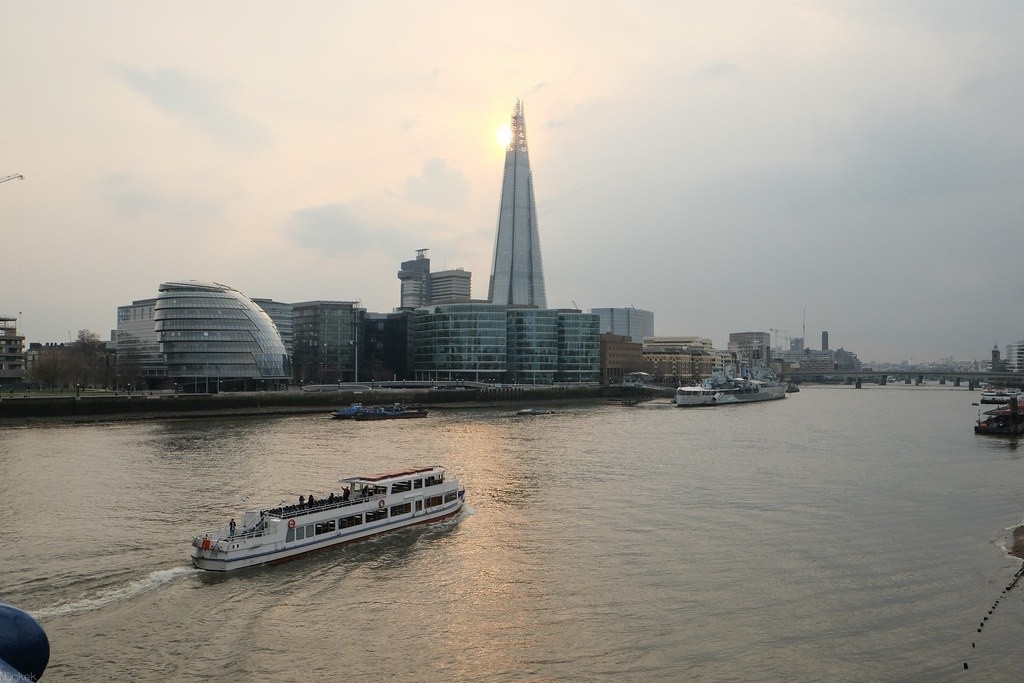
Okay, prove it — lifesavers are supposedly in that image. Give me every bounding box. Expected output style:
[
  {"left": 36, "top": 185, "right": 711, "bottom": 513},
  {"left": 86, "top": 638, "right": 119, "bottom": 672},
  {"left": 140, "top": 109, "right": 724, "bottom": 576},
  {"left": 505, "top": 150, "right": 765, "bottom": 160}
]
[
  {"left": 287, "top": 519, "right": 296, "bottom": 529},
  {"left": 379, "top": 499, "right": 385, "bottom": 508}
]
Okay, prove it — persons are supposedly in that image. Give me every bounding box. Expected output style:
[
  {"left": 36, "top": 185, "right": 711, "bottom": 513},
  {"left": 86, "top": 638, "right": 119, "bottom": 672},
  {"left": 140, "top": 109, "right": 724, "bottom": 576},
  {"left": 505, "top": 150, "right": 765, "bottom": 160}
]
[
  {"left": 229, "top": 519, "right": 236, "bottom": 535},
  {"left": 299, "top": 495, "right": 305, "bottom": 510},
  {"left": 341, "top": 486, "right": 350, "bottom": 501},
  {"left": 363, "top": 485, "right": 369, "bottom": 493},
  {"left": 308, "top": 495, "right": 314, "bottom": 508}
]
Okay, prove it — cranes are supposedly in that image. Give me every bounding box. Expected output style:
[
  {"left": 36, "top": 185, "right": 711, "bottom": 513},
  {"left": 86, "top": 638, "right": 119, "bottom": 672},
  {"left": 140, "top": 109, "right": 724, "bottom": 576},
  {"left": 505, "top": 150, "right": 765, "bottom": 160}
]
[{"left": 769, "top": 329, "right": 787, "bottom": 350}]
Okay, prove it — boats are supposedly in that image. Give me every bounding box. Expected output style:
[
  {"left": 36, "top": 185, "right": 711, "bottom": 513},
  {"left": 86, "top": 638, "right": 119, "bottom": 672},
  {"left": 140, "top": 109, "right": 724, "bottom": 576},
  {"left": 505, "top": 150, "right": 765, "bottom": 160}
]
[
  {"left": 676, "top": 353, "right": 790, "bottom": 406},
  {"left": 189, "top": 464, "right": 466, "bottom": 572},
  {"left": 975, "top": 387, "right": 1024, "bottom": 440},
  {"left": 331, "top": 405, "right": 428, "bottom": 420},
  {"left": 518, "top": 408, "right": 550, "bottom": 415}
]
